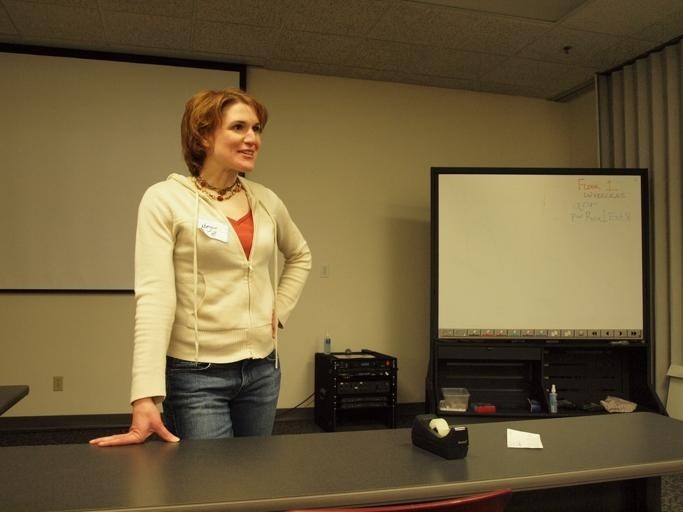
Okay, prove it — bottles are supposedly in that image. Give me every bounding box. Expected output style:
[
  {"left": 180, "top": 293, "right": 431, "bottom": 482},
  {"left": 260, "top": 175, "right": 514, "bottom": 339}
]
[
  {"left": 324, "top": 335, "right": 331, "bottom": 355},
  {"left": 547, "top": 383, "right": 558, "bottom": 413}
]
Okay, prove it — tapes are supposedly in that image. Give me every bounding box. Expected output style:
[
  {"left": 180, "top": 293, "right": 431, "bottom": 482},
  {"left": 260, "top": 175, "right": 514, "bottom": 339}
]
[{"left": 429, "top": 418, "right": 450, "bottom": 438}]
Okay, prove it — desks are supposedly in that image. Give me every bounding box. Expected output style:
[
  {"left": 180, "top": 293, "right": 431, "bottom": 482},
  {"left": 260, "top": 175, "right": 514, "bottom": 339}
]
[{"left": 0, "top": 410, "right": 683, "bottom": 511}]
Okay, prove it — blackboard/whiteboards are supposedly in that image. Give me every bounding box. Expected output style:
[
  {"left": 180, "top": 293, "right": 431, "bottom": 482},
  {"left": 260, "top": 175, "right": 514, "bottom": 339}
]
[{"left": 0, "top": 43, "right": 246, "bottom": 293}]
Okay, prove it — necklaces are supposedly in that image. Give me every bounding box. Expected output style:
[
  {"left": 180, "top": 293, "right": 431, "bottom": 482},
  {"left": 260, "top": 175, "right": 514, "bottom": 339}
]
[{"left": 194, "top": 171, "right": 242, "bottom": 202}]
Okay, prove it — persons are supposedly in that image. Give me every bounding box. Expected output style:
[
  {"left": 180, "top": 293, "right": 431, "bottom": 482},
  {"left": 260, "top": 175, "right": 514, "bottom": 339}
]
[{"left": 87, "top": 87, "right": 313, "bottom": 447}]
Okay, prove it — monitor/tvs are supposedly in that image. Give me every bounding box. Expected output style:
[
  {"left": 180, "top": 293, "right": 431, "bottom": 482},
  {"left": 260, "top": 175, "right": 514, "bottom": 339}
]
[{"left": 431, "top": 167, "right": 650, "bottom": 347}]
